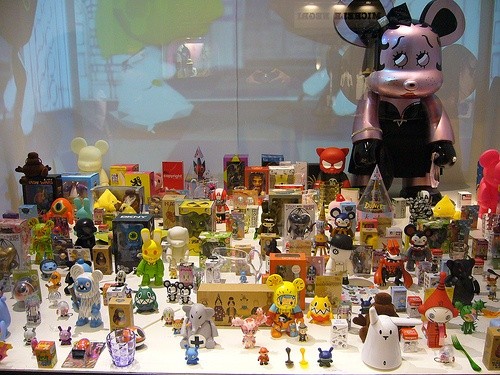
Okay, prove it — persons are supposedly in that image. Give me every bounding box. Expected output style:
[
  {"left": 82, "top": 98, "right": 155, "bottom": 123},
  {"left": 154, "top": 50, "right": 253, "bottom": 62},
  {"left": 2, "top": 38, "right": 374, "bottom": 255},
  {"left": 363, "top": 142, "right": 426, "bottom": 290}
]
[{"left": 252, "top": 175, "right": 264, "bottom": 194}]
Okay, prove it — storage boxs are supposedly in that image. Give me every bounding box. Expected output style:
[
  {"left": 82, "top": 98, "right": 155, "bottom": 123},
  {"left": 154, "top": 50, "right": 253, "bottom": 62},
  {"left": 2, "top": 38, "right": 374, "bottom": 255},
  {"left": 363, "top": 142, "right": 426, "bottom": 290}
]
[{"left": 0, "top": 153, "right": 500, "bottom": 371}]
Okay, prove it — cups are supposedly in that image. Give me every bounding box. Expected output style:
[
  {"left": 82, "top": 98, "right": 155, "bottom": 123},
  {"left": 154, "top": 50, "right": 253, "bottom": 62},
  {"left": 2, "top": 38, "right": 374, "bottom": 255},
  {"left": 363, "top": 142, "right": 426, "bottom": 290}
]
[
  {"left": 106, "top": 329, "right": 134, "bottom": 365},
  {"left": 212, "top": 247, "right": 249, "bottom": 283},
  {"left": 450, "top": 242, "right": 469, "bottom": 261}
]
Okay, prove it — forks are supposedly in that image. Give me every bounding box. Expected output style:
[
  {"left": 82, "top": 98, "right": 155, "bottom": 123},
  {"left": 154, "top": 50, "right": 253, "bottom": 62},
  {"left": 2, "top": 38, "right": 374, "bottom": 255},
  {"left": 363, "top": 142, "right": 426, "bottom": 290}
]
[{"left": 451, "top": 333, "right": 481, "bottom": 371}]
